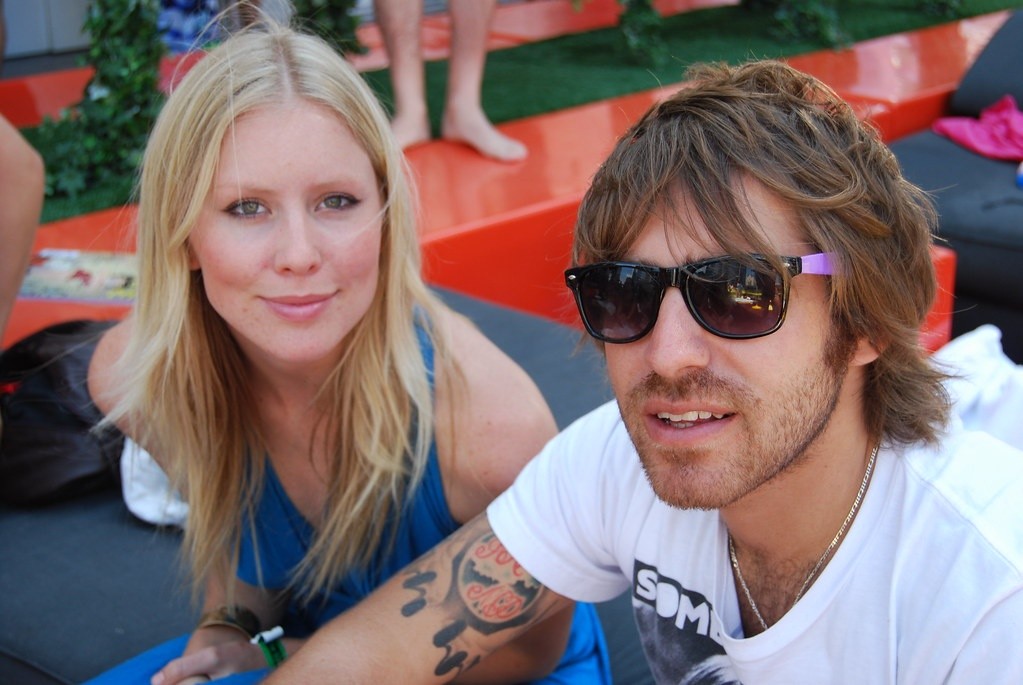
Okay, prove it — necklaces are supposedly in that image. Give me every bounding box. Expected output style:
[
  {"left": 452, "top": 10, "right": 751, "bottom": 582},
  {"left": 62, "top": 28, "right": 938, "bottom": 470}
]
[{"left": 728, "top": 446, "right": 878, "bottom": 630}]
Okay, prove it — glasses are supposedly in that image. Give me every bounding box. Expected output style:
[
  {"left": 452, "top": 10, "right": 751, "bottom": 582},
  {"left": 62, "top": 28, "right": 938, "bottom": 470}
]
[{"left": 564, "top": 250, "right": 838, "bottom": 344}]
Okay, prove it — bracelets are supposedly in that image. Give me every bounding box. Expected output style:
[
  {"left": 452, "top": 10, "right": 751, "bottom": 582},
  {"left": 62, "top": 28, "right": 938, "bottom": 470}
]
[
  {"left": 198, "top": 605, "right": 261, "bottom": 640},
  {"left": 253, "top": 626, "right": 288, "bottom": 668}
]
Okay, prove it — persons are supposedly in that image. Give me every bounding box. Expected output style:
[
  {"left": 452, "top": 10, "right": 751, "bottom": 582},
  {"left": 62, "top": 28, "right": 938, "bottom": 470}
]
[
  {"left": 76, "top": 30, "right": 609, "bottom": 685},
  {"left": 373, "top": 0, "right": 526, "bottom": 164},
  {"left": 0, "top": 116, "right": 47, "bottom": 346},
  {"left": 262, "top": 55, "right": 1023, "bottom": 685}
]
